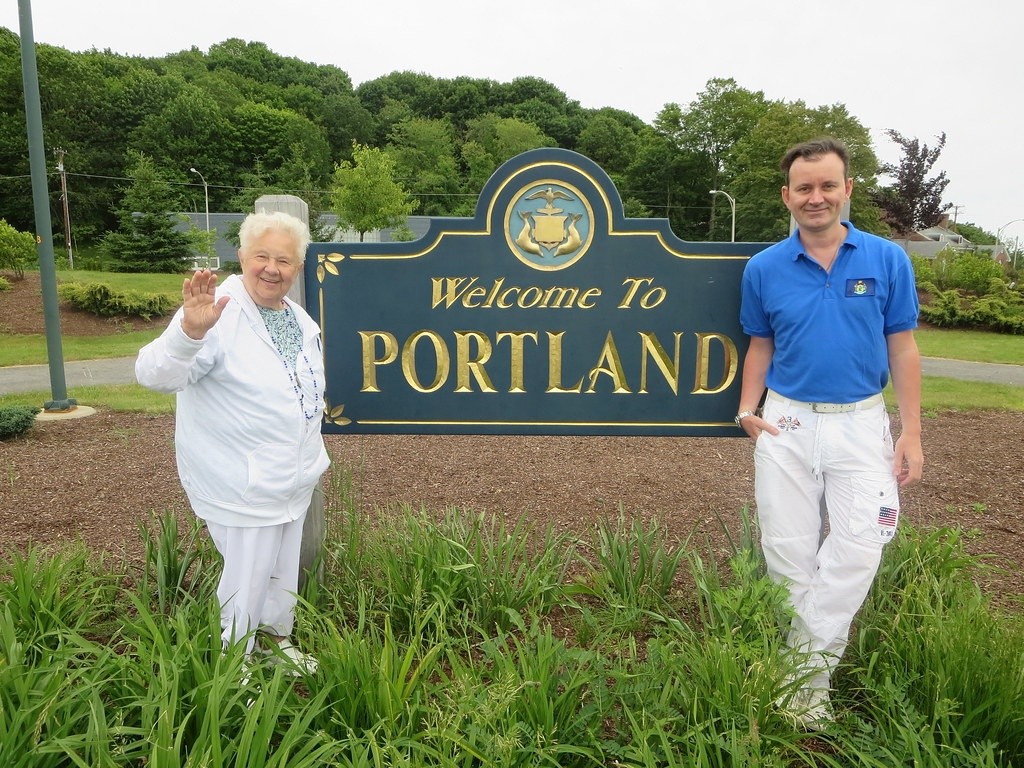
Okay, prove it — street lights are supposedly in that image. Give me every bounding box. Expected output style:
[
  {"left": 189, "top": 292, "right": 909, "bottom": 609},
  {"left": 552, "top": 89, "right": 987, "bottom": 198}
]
[
  {"left": 190, "top": 167, "right": 210, "bottom": 257},
  {"left": 709, "top": 189, "right": 737, "bottom": 243}
]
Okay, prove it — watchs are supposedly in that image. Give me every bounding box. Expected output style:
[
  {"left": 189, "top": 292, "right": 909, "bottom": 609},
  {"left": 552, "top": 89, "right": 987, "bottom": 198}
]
[{"left": 734, "top": 411, "right": 753, "bottom": 428}]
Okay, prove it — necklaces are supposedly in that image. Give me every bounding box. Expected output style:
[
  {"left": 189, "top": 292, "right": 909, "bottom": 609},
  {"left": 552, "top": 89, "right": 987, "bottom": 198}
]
[
  {"left": 260, "top": 299, "right": 318, "bottom": 419},
  {"left": 826, "top": 224, "right": 841, "bottom": 271}
]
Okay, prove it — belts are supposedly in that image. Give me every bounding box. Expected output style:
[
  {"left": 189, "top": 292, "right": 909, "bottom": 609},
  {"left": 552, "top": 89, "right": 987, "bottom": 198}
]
[{"left": 768, "top": 389, "right": 880, "bottom": 412}]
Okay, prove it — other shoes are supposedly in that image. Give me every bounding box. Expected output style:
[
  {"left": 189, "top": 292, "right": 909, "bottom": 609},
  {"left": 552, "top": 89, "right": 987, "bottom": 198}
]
[
  {"left": 254, "top": 633, "right": 319, "bottom": 677},
  {"left": 773, "top": 691, "right": 833, "bottom": 733},
  {"left": 238, "top": 665, "right": 263, "bottom": 711}
]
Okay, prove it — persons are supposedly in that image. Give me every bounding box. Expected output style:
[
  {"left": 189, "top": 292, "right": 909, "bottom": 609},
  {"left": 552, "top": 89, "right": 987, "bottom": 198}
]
[
  {"left": 134, "top": 209, "right": 330, "bottom": 711},
  {"left": 730, "top": 137, "right": 923, "bottom": 741}
]
